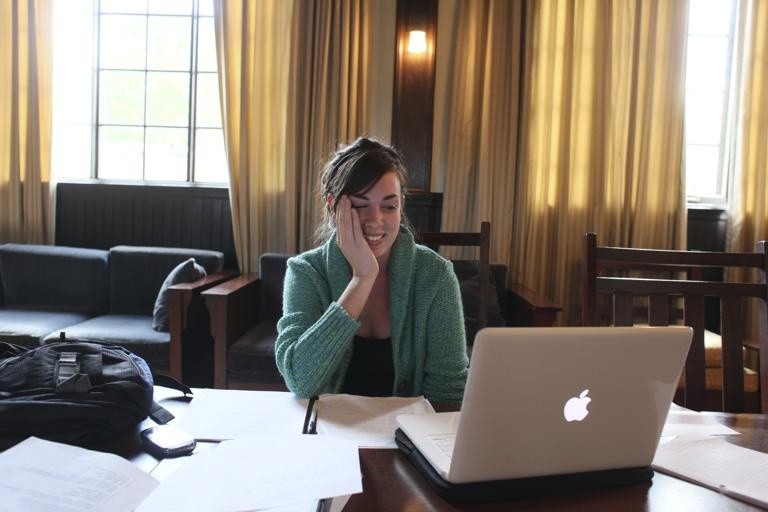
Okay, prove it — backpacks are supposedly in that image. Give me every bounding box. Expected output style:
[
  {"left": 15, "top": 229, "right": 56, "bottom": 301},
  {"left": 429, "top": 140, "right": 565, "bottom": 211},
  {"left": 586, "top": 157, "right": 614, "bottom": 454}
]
[{"left": 0, "top": 329, "right": 153, "bottom": 437}]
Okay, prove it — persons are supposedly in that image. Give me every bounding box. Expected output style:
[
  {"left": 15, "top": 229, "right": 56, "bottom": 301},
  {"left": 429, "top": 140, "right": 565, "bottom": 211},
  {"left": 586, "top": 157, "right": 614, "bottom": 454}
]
[{"left": 275, "top": 136, "right": 468, "bottom": 406}]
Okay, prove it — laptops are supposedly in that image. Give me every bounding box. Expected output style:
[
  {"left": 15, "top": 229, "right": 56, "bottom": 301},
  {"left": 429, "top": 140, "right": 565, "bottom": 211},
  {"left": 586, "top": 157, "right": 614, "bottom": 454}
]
[{"left": 394, "top": 327, "right": 693, "bottom": 505}]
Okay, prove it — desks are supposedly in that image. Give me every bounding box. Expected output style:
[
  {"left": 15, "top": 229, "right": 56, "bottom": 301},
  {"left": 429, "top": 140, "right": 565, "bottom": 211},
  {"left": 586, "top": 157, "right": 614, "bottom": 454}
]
[{"left": 0, "top": 383, "right": 768, "bottom": 512}]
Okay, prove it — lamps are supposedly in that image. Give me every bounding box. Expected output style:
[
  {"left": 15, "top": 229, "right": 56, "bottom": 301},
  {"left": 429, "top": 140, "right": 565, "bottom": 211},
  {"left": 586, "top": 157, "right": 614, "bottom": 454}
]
[{"left": 405, "top": 7, "right": 427, "bottom": 55}]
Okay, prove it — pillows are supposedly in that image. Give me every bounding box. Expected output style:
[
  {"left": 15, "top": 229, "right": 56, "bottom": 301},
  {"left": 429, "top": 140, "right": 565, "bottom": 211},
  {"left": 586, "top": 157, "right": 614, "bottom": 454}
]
[{"left": 450, "top": 257, "right": 508, "bottom": 337}]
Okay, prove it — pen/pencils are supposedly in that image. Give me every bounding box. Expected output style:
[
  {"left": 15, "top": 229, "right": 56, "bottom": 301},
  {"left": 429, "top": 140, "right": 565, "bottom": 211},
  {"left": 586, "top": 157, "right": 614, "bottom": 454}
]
[{"left": 307, "top": 399, "right": 318, "bottom": 433}]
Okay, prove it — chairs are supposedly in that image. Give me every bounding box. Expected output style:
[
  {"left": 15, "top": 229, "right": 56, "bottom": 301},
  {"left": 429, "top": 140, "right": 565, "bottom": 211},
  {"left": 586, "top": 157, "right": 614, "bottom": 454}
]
[
  {"left": 409, "top": 220, "right": 492, "bottom": 344},
  {"left": 581, "top": 229, "right": 768, "bottom": 416}
]
